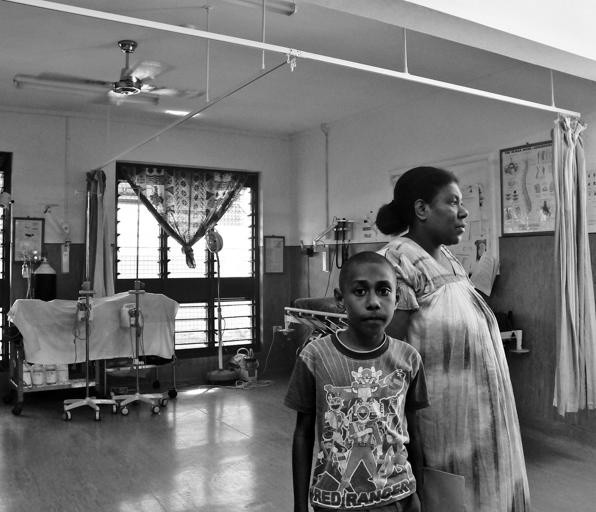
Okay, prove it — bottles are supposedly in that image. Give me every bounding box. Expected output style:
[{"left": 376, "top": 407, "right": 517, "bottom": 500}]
[
  {"left": 46, "top": 364, "right": 57, "bottom": 385},
  {"left": 24, "top": 367, "right": 32, "bottom": 386},
  {"left": 31, "top": 363, "right": 43, "bottom": 386}
]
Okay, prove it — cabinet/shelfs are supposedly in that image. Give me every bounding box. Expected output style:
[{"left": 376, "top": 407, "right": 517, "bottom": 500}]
[{"left": 11, "top": 299, "right": 178, "bottom": 416}]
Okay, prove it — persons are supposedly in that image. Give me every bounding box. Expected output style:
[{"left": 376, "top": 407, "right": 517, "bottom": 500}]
[
  {"left": 283, "top": 251, "right": 431, "bottom": 512},
  {"left": 374, "top": 167, "right": 530, "bottom": 512}
]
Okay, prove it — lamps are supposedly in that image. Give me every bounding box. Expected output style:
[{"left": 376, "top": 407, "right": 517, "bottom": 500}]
[
  {"left": 12, "top": 75, "right": 96, "bottom": 95},
  {"left": 204, "top": 226, "right": 237, "bottom": 385},
  {"left": 241, "top": 0, "right": 297, "bottom": 15}
]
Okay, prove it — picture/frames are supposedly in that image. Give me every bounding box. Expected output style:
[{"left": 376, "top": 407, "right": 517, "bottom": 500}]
[
  {"left": 13, "top": 217, "right": 45, "bottom": 263},
  {"left": 500, "top": 140, "right": 561, "bottom": 237},
  {"left": 263, "top": 235, "right": 286, "bottom": 276}
]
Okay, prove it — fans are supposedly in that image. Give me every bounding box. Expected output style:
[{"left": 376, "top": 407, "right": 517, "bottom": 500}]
[{"left": 40, "top": 39, "right": 204, "bottom": 106}]
